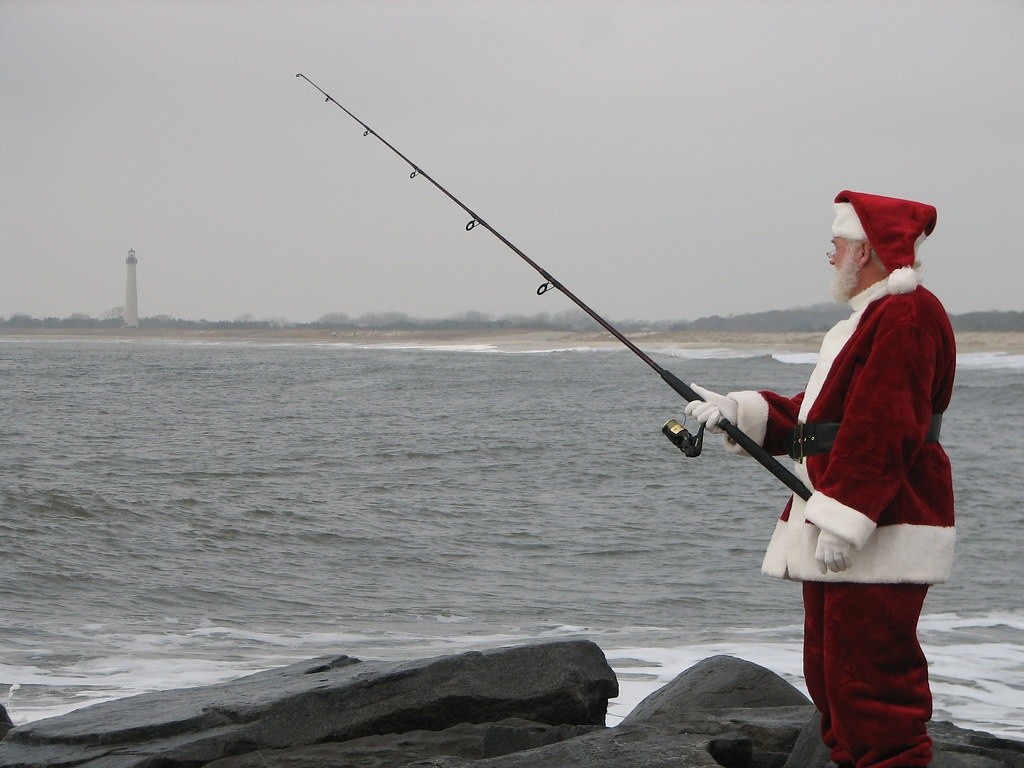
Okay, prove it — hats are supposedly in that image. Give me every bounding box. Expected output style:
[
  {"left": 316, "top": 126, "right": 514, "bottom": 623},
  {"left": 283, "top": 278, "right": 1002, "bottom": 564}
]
[{"left": 832, "top": 190, "right": 937, "bottom": 295}]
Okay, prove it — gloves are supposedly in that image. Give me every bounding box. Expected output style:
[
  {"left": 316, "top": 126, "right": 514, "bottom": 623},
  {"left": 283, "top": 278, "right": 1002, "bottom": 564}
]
[
  {"left": 815, "top": 530, "right": 852, "bottom": 574},
  {"left": 685, "top": 383, "right": 738, "bottom": 434}
]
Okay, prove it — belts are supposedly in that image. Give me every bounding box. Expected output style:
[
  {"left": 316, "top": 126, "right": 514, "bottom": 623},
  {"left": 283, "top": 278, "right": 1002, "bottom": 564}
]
[{"left": 789, "top": 413, "right": 943, "bottom": 464}]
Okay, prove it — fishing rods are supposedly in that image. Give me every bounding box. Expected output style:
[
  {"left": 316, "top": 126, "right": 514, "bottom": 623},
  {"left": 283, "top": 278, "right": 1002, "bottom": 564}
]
[{"left": 297, "top": 71, "right": 815, "bottom": 503}]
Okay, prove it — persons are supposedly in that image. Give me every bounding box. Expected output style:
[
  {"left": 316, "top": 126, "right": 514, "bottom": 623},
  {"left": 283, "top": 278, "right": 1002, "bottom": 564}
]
[{"left": 685, "top": 190, "right": 957, "bottom": 768}]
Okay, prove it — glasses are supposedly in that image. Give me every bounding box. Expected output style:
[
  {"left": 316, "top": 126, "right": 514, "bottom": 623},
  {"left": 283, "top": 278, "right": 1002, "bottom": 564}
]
[{"left": 826, "top": 245, "right": 847, "bottom": 259}]
